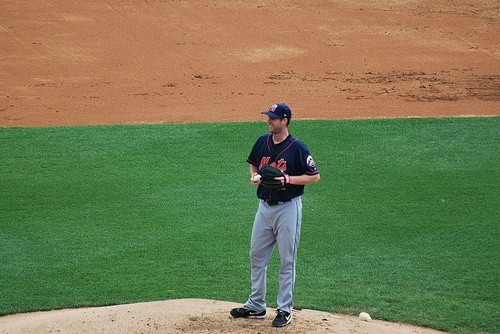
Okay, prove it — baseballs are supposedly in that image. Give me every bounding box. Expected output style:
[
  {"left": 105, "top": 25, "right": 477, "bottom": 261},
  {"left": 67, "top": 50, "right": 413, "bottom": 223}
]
[{"left": 253, "top": 174, "right": 262, "bottom": 183}]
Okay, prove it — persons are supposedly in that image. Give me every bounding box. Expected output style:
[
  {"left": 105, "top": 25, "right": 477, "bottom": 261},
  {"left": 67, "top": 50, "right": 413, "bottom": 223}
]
[{"left": 230, "top": 102, "right": 321, "bottom": 327}]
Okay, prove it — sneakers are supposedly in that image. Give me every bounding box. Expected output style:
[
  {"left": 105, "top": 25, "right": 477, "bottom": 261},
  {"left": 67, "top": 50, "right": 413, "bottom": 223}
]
[
  {"left": 231, "top": 307, "right": 267, "bottom": 319},
  {"left": 272, "top": 309, "right": 291, "bottom": 327}
]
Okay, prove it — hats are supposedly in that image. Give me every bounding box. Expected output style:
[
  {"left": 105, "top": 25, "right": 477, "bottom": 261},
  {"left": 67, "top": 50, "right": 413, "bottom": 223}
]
[{"left": 261, "top": 103, "right": 293, "bottom": 119}]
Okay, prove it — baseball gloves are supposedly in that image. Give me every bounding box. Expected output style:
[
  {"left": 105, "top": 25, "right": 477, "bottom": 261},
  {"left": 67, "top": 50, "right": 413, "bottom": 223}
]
[{"left": 258, "top": 165, "right": 286, "bottom": 188}]
